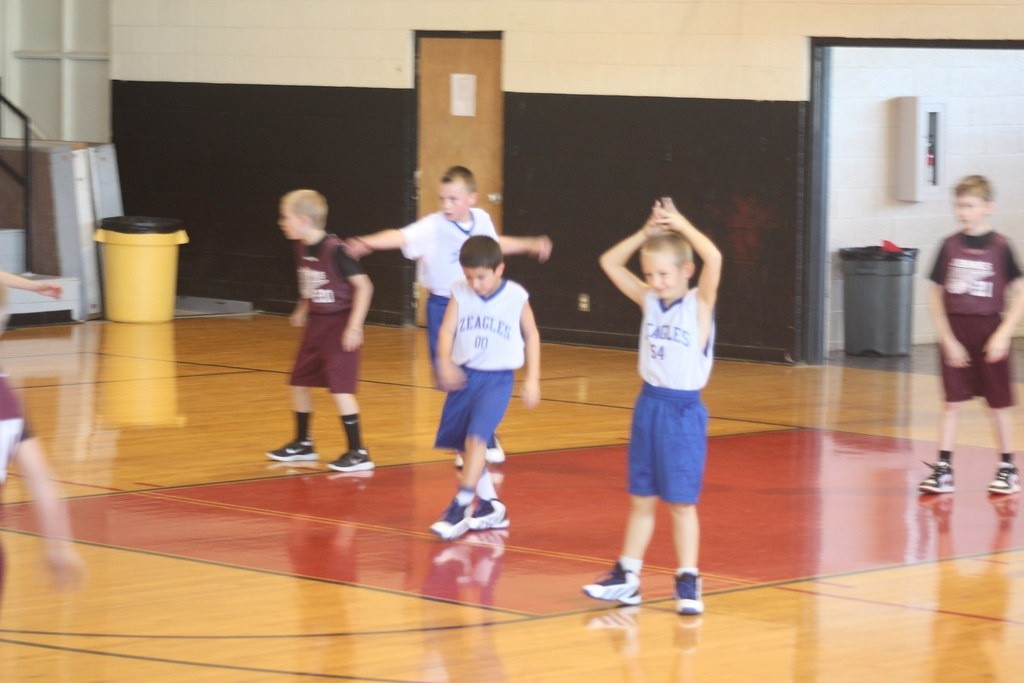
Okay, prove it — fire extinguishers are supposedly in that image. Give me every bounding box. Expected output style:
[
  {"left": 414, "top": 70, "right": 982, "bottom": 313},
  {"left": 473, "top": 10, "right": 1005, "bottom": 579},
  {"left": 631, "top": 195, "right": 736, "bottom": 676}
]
[{"left": 926, "top": 134, "right": 935, "bottom": 185}]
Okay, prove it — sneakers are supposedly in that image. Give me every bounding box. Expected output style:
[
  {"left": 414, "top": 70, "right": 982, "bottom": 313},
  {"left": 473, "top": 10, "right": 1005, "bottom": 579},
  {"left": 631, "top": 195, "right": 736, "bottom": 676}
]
[
  {"left": 581, "top": 560, "right": 641, "bottom": 604},
  {"left": 988, "top": 462, "right": 1021, "bottom": 493},
  {"left": 326, "top": 448, "right": 375, "bottom": 471},
  {"left": 484, "top": 433, "right": 505, "bottom": 463},
  {"left": 674, "top": 572, "right": 703, "bottom": 614},
  {"left": 266, "top": 437, "right": 319, "bottom": 461},
  {"left": 470, "top": 498, "right": 510, "bottom": 530},
  {"left": 917, "top": 461, "right": 955, "bottom": 492},
  {"left": 430, "top": 495, "right": 479, "bottom": 540},
  {"left": 455, "top": 453, "right": 464, "bottom": 466}
]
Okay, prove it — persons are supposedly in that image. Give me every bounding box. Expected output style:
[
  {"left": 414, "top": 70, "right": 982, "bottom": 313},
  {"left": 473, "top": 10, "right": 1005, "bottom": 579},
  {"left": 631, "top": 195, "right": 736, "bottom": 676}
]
[
  {"left": 0, "top": 269, "right": 84, "bottom": 613},
  {"left": 917, "top": 175, "right": 1024, "bottom": 495},
  {"left": 582, "top": 197, "right": 723, "bottom": 616},
  {"left": 341, "top": 165, "right": 553, "bottom": 467},
  {"left": 430, "top": 234, "right": 542, "bottom": 539},
  {"left": 264, "top": 188, "right": 375, "bottom": 473}
]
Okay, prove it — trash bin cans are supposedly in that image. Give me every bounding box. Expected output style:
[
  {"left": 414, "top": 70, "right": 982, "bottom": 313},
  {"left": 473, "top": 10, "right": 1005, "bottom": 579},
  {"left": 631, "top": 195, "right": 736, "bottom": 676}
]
[
  {"left": 92, "top": 215, "right": 190, "bottom": 323},
  {"left": 838, "top": 246, "right": 919, "bottom": 356}
]
